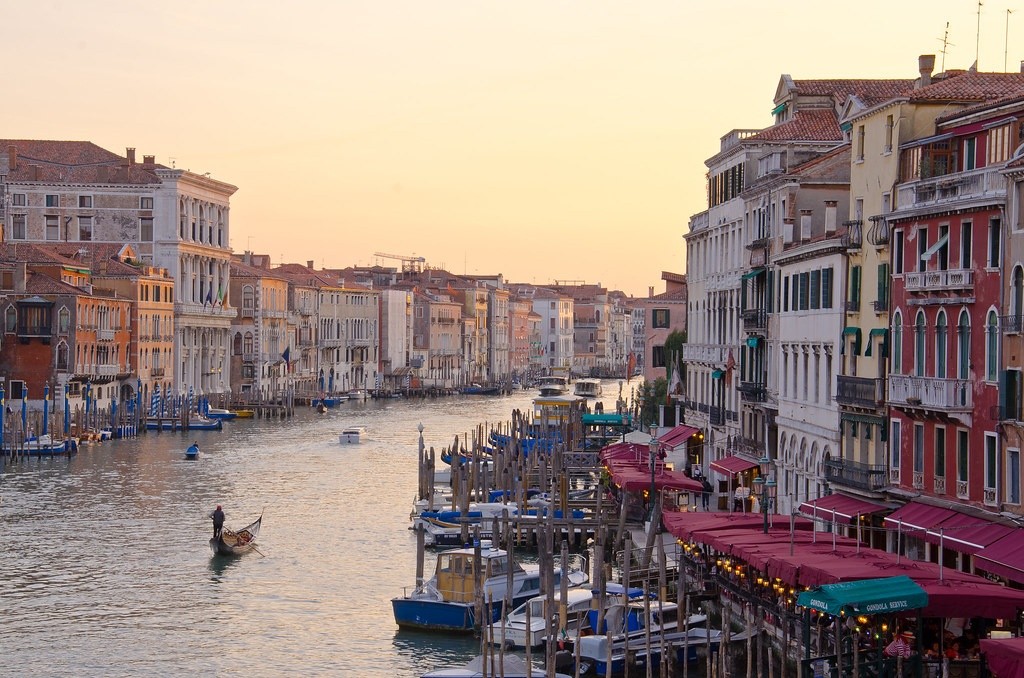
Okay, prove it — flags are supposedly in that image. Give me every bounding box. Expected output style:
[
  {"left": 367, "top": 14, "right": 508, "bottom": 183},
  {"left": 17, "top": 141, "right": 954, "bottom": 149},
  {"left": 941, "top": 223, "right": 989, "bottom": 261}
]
[
  {"left": 725, "top": 349, "right": 735, "bottom": 387},
  {"left": 627, "top": 352, "right": 636, "bottom": 383},
  {"left": 282, "top": 346, "right": 290, "bottom": 373},
  {"left": 207, "top": 286, "right": 229, "bottom": 311}
]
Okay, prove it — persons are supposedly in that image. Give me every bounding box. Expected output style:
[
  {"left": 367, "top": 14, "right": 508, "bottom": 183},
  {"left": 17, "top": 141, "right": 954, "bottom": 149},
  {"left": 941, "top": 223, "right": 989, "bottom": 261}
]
[
  {"left": 694, "top": 465, "right": 701, "bottom": 481},
  {"left": 209, "top": 505, "right": 226, "bottom": 538},
  {"left": 680, "top": 542, "right": 980, "bottom": 677},
  {"left": 734, "top": 484, "right": 743, "bottom": 511},
  {"left": 684, "top": 461, "right": 692, "bottom": 478},
  {"left": 193, "top": 441, "right": 198, "bottom": 448},
  {"left": 6, "top": 404, "right": 13, "bottom": 414},
  {"left": 702, "top": 477, "right": 714, "bottom": 510}
]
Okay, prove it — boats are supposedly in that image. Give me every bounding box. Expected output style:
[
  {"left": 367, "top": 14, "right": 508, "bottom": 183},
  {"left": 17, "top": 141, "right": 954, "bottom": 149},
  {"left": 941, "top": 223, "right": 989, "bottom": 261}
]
[
  {"left": 390, "top": 539, "right": 589, "bottom": 636},
  {"left": 573, "top": 377, "right": 602, "bottom": 397},
  {"left": 411, "top": 426, "right": 599, "bottom": 546},
  {"left": 531, "top": 365, "right": 586, "bottom": 426},
  {"left": 463, "top": 387, "right": 499, "bottom": 394},
  {"left": 144, "top": 405, "right": 255, "bottom": 430},
  {"left": 185, "top": 446, "right": 201, "bottom": 461},
  {"left": 339, "top": 426, "right": 368, "bottom": 445},
  {"left": 419, "top": 652, "right": 572, "bottom": 678},
  {"left": 0, "top": 423, "right": 137, "bottom": 454},
  {"left": 348, "top": 389, "right": 372, "bottom": 399},
  {"left": 487, "top": 583, "right": 737, "bottom": 678},
  {"left": 217, "top": 507, "right": 265, "bottom": 554},
  {"left": 311, "top": 396, "right": 350, "bottom": 412}
]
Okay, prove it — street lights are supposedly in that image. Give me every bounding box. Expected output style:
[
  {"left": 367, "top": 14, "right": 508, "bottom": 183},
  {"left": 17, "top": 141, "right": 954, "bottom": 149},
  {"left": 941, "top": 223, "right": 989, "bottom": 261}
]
[
  {"left": 647, "top": 421, "right": 659, "bottom": 522},
  {"left": 620, "top": 401, "right": 629, "bottom": 443},
  {"left": 751, "top": 456, "right": 777, "bottom": 534}
]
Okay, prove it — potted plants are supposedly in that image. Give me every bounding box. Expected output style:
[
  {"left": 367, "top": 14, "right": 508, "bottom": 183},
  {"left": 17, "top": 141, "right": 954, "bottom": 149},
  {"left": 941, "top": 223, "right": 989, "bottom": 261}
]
[{"left": 906, "top": 396, "right": 921, "bottom": 406}]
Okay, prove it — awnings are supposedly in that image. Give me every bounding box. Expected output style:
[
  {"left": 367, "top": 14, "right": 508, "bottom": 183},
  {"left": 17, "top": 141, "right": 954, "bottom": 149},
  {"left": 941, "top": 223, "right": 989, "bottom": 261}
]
[
  {"left": 925, "top": 512, "right": 1017, "bottom": 554},
  {"left": 974, "top": 528, "right": 1024, "bottom": 587},
  {"left": 657, "top": 425, "right": 690, "bottom": 451},
  {"left": 883, "top": 500, "right": 958, "bottom": 540},
  {"left": 709, "top": 454, "right": 760, "bottom": 479},
  {"left": 798, "top": 490, "right": 901, "bottom": 523},
  {"left": 663, "top": 427, "right": 700, "bottom": 451}
]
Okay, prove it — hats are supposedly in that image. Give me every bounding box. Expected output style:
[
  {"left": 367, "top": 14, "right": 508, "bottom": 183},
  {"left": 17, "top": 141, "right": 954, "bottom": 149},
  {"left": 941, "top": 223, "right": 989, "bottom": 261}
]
[{"left": 217, "top": 504, "right": 222, "bottom": 509}]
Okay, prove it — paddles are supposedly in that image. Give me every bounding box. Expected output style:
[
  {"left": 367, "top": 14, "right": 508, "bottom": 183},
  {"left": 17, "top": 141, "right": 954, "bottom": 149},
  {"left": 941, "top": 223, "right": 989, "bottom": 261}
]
[{"left": 211, "top": 517, "right": 265, "bottom": 557}]
